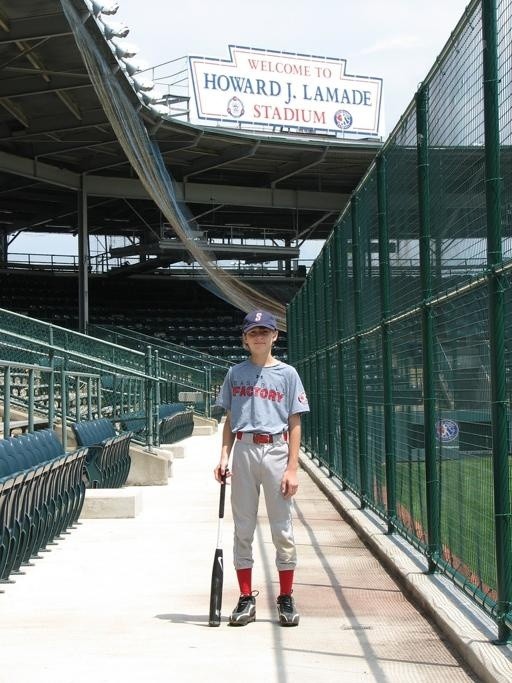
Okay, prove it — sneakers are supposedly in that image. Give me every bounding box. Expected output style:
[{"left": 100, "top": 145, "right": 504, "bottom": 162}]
[
  {"left": 229, "top": 596, "right": 256, "bottom": 625},
  {"left": 276, "top": 595, "right": 299, "bottom": 625}
]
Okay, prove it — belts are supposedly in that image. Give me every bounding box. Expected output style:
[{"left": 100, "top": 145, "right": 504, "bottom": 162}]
[{"left": 237, "top": 431, "right": 287, "bottom": 444}]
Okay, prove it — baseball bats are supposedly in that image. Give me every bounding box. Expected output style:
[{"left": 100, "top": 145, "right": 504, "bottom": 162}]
[{"left": 209, "top": 468, "right": 229, "bottom": 627}]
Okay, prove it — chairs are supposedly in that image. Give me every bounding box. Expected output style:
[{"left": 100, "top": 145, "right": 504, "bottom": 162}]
[
  {"left": 76, "top": 416, "right": 134, "bottom": 488},
  {"left": 41, "top": 354, "right": 193, "bottom": 445},
  {"left": 196, "top": 398, "right": 226, "bottom": 423},
  {"left": 0, "top": 427, "right": 89, "bottom": 584},
  {"left": 0, "top": 283, "right": 287, "bottom": 384}
]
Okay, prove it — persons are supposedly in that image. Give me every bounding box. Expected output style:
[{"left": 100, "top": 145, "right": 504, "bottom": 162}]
[{"left": 215, "top": 310, "right": 310, "bottom": 626}]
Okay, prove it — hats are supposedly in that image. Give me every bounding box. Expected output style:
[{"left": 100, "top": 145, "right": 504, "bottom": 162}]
[{"left": 242, "top": 310, "right": 277, "bottom": 333}]
